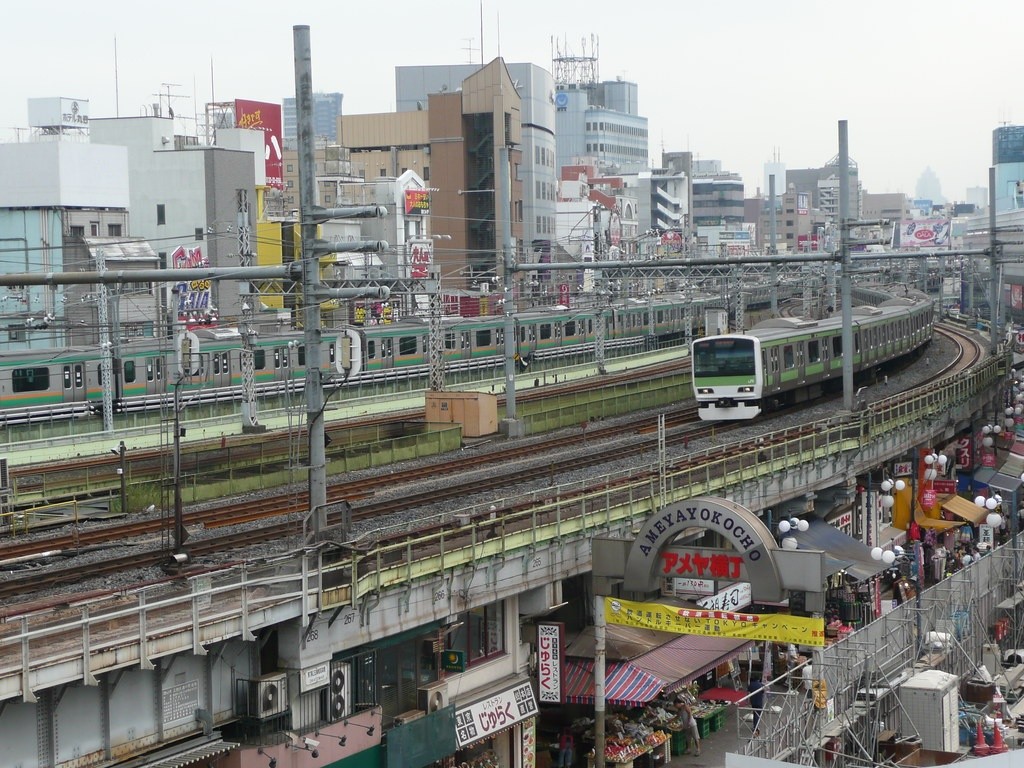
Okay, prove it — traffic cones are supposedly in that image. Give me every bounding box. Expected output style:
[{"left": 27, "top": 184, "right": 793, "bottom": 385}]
[{"left": 973, "top": 718, "right": 1010, "bottom": 757}]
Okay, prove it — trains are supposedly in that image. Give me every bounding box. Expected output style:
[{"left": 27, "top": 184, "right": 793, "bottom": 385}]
[
  {"left": 0, "top": 293, "right": 736, "bottom": 423},
  {"left": 688, "top": 287, "right": 938, "bottom": 421}
]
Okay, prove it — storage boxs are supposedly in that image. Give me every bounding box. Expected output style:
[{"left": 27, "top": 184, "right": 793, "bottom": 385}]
[{"left": 665, "top": 706, "right": 726, "bottom": 756}]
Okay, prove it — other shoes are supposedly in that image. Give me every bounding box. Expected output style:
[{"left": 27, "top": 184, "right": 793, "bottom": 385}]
[
  {"left": 695, "top": 750, "right": 701, "bottom": 756},
  {"left": 682, "top": 748, "right": 691, "bottom": 753}
]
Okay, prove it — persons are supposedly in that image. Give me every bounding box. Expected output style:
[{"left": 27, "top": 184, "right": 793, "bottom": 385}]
[
  {"left": 802, "top": 656, "right": 819, "bottom": 713},
  {"left": 831, "top": 615, "right": 842, "bottom": 627},
  {"left": 669, "top": 698, "right": 701, "bottom": 756},
  {"left": 784, "top": 643, "right": 801, "bottom": 687},
  {"left": 746, "top": 674, "right": 769, "bottom": 731},
  {"left": 954, "top": 501, "right": 1024, "bottom": 572},
  {"left": 557, "top": 723, "right": 575, "bottom": 768}
]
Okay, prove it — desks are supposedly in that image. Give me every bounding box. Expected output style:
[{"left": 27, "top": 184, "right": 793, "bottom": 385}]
[{"left": 584, "top": 735, "right": 672, "bottom": 768}]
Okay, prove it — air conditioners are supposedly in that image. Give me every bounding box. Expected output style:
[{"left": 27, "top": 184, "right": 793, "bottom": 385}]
[
  {"left": 322, "top": 662, "right": 351, "bottom": 722},
  {"left": 247, "top": 672, "right": 288, "bottom": 718},
  {"left": 417, "top": 680, "right": 448, "bottom": 713},
  {"left": 392, "top": 709, "right": 425, "bottom": 727}
]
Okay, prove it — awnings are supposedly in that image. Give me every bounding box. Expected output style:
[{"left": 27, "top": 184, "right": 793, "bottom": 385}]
[
  {"left": 942, "top": 496, "right": 990, "bottom": 528},
  {"left": 564, "top": 635, "right": 754, "bottom": 708}
]
